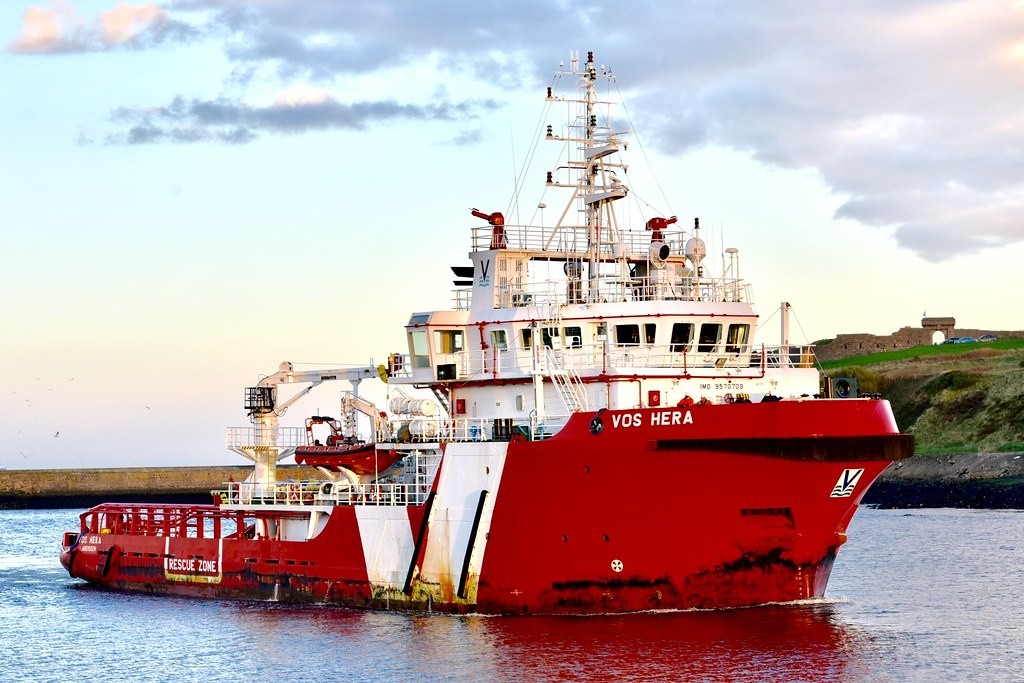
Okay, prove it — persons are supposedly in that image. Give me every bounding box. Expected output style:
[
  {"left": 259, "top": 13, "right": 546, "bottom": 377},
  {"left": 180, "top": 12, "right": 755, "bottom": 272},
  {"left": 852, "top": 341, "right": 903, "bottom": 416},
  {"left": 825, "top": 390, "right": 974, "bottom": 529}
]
[
  {"left": 676, "top": 392, "right": 712, "bottom": 407},
  {"left": 315, "top": 440, "right": 324, "bottom": 447}
]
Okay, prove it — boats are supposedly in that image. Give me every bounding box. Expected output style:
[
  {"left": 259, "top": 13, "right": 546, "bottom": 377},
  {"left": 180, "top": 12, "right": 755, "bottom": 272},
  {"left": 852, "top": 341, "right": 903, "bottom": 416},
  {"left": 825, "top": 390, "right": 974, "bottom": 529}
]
[{"left": 59, "top": 51, "right": 914, "bottom": 619}]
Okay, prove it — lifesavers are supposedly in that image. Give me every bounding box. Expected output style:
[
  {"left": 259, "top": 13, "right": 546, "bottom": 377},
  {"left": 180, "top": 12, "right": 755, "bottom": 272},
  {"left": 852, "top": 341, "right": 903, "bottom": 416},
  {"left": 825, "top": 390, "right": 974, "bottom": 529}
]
[
  {"left": 290, "top": 485, "right": 301, "bottom": 500},
  {"left": 371, "top": 486, "right": 382, "bottom": 501}
]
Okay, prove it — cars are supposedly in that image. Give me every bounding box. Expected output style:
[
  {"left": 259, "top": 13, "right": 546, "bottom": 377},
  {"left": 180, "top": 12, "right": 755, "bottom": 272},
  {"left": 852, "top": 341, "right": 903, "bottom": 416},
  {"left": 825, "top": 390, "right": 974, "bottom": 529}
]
[{"left": 942, "top": 334, "right": 997, "bottom": 344}]
